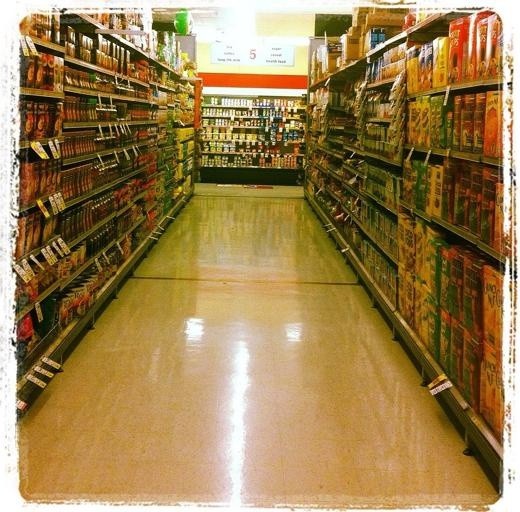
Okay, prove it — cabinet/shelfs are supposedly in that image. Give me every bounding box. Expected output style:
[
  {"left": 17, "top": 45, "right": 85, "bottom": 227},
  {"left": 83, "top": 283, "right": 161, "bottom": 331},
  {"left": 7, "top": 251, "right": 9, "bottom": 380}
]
[{"left": 201, "top": 92, "right": 307, "bottom": 184}]
